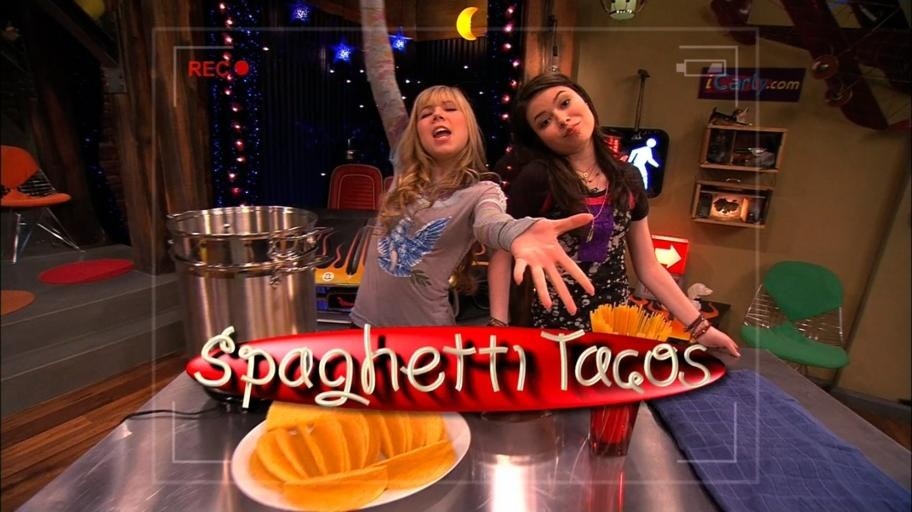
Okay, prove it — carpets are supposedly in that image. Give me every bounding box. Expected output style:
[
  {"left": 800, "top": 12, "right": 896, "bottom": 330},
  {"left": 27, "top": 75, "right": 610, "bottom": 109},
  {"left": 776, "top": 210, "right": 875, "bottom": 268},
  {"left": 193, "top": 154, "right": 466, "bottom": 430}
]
[
  {"left": 0, "top": 287, "right": 37, "bottom": 315},
  {"left": 36, "top": 257, "right": 137, "bottom": 286}
]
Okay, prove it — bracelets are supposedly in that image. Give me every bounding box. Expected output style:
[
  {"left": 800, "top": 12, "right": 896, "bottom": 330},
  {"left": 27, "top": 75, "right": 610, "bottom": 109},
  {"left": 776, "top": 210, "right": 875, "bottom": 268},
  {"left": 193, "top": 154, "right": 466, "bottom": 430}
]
[
  {"left": 684, "top": 313, "right": 713, "bottom": 341},
  {"left": 487, "top": 317, "right": 506, "bottom": 326}
]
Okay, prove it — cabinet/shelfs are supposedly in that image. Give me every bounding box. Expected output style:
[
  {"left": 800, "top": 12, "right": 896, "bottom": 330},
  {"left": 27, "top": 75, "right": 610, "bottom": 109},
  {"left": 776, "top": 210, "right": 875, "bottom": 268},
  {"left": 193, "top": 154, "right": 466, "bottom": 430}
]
[{"left": 690, "top": 118, "right": 789, "bottom": 232}]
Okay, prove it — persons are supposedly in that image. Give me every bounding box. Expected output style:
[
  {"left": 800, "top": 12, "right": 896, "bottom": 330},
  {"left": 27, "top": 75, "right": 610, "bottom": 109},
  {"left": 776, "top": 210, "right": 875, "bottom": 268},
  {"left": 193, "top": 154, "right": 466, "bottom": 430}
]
[
  {"left": 483, "top": 71, "right": 743, "bottom": 357},
  {"left": 347, "top": 0, "right": 595, "bottom": 330}
]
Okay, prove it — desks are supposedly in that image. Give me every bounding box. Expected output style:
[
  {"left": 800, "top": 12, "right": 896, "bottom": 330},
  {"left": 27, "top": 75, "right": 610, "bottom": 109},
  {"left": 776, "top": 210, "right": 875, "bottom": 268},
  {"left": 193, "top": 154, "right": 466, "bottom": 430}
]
[{"left": 9, "top": 334, "right": 912, "bottom": 510}]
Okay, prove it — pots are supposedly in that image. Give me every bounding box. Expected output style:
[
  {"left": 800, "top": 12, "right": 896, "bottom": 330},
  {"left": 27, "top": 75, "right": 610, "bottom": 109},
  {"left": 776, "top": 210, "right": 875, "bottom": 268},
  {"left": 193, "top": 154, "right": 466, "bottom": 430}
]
[{"left": 161, "top": 204, "right": 335, "bottom": 365}]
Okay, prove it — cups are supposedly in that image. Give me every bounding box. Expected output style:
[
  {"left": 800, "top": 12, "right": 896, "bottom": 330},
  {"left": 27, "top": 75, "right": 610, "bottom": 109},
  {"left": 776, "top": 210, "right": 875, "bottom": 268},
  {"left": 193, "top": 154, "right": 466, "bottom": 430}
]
[
  {"left": 587, "top": 401, "right": 640, "bottom": 457},
  {"left": 583, "top": 453, "right": 623, "bottom": 511}
]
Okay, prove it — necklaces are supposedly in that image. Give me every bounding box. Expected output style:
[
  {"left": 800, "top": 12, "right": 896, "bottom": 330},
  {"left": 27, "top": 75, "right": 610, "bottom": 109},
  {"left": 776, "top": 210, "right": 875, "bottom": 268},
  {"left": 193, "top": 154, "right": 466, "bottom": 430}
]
[{"left": 571, "top": 159, "right": 611, "bottom": 244}]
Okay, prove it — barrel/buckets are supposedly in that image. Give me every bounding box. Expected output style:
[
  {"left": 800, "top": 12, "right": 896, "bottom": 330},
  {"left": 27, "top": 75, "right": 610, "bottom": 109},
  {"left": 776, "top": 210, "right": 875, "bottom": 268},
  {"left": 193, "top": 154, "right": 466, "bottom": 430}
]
[{"left": 165, "top": 205, "right": 336, "bottom": 361}]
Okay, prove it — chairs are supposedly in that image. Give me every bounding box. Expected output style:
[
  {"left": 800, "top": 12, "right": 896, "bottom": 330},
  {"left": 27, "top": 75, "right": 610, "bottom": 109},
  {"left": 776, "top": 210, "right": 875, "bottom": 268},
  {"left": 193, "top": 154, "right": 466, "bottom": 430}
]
[
  {"left": 1, "top": 143, "right": 89, "bottom": 264},
  {"left": 313, "top": 164, "right": 514, "bottom": 315},
  {"left": 738, "top": 258, "right": 849, "bottom": 379}
]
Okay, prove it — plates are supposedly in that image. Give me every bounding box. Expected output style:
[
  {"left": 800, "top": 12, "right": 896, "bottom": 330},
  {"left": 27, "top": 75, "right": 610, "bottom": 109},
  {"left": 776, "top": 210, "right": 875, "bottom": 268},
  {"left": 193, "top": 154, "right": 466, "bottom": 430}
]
[{"left": 229, "top": 411, "right": 470, "bottom": 512}]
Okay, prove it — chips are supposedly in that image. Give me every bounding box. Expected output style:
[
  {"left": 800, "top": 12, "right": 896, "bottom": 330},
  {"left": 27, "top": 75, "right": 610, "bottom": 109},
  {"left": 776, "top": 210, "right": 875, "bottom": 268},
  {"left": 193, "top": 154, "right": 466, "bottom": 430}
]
[{"left": 250, "top": 400, "right": 458, "bottom": 511}]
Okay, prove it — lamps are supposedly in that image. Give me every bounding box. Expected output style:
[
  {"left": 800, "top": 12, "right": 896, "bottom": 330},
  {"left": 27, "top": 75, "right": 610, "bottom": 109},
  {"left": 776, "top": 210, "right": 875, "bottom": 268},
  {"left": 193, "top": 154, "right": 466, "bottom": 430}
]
[{"left": 598, "top": 0, "right": 646, "bottom": 20}]
